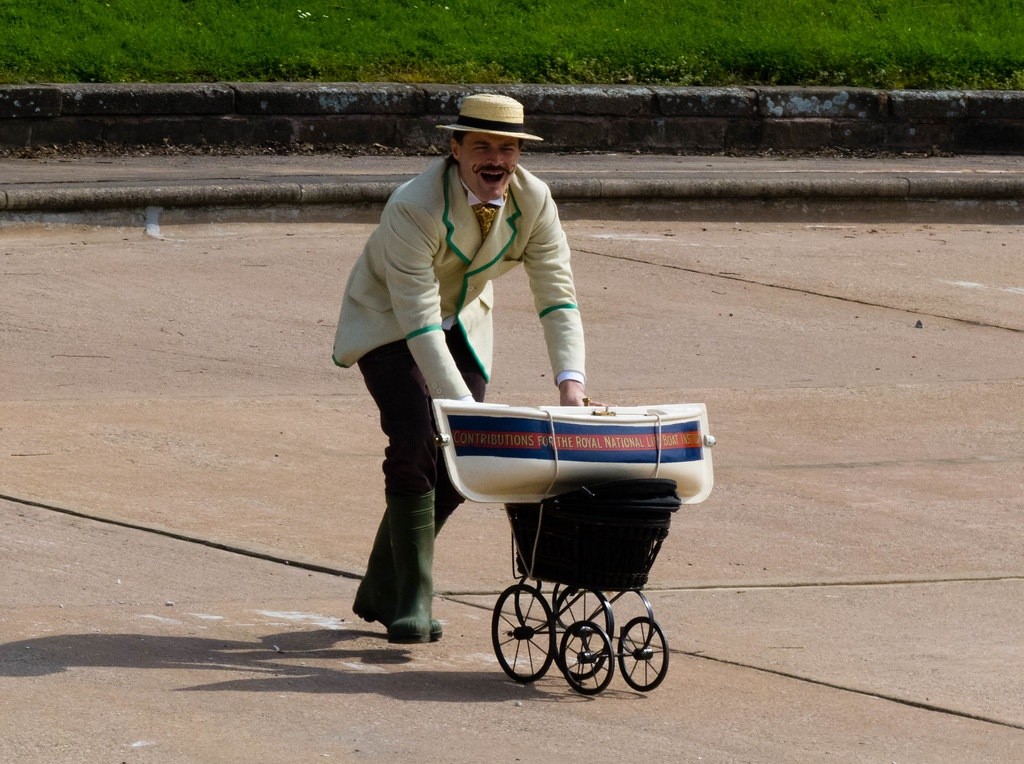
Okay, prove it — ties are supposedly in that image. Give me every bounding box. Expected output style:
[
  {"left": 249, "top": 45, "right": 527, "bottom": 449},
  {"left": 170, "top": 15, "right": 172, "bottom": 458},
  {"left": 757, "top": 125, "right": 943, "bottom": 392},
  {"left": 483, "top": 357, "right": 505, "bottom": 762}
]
[{"left": 474, "top": 204, "right": 500, "bottom": 240}]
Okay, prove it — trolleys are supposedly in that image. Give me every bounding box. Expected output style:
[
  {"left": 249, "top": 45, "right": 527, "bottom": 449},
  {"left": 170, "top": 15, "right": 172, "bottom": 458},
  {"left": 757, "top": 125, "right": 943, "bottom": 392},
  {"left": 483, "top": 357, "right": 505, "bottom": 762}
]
[{"left": 492, "top": 476, "right": 680, "bottom": 695}]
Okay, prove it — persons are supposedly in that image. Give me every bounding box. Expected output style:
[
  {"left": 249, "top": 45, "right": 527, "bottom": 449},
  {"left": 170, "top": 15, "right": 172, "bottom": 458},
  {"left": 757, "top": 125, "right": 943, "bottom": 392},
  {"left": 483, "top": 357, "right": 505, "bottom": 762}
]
[{"left": 331, "top": 91, "right": 609, "bottom": 648}]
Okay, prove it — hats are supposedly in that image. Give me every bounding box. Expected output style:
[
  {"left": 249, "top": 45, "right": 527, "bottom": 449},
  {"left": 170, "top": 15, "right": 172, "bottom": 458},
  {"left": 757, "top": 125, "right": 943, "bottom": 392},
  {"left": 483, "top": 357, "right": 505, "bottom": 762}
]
[{"left": 437, "top": 93, "right": 544, "bottom": 141}]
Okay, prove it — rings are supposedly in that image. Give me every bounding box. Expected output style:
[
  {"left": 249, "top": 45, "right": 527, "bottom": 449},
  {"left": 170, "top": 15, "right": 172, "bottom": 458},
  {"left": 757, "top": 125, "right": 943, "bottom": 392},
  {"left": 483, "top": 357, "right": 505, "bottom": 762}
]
[{"left": 583, "top": 397, "right": 591, "bottom": 407}]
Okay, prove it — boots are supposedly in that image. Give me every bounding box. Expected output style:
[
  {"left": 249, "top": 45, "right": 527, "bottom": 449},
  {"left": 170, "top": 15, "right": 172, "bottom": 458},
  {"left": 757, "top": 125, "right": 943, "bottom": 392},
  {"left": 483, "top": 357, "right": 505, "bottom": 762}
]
[
  {"left": 352, "top": 505, "right": 442, "bottom": 639},
  {"left": 386, "top": 489, "right": 435, "bottom": 643}
]
